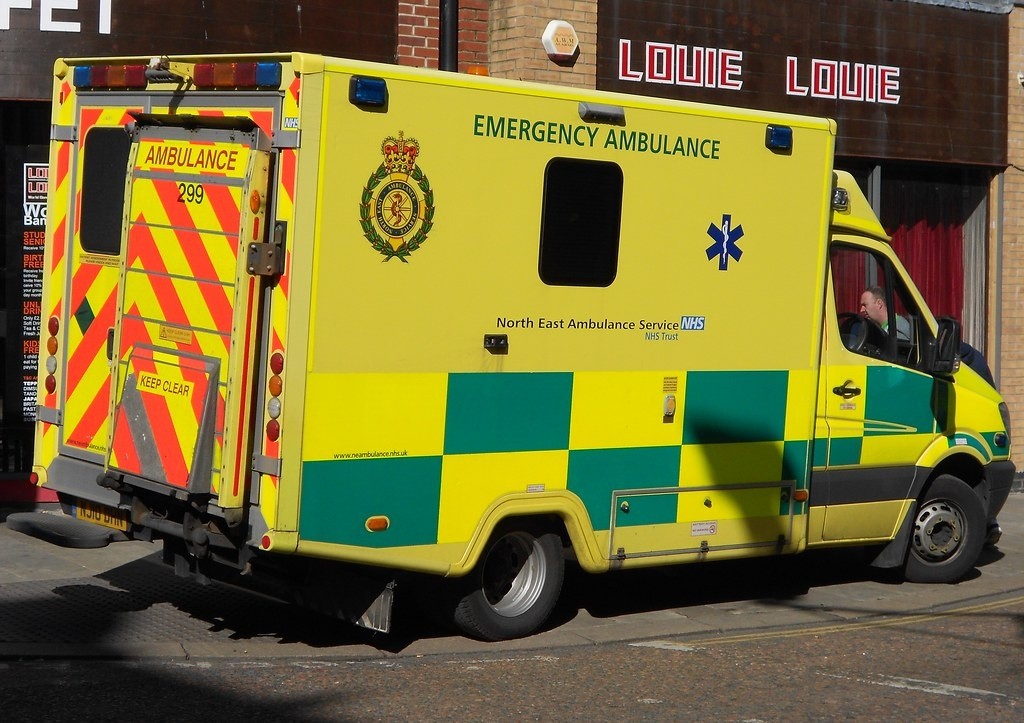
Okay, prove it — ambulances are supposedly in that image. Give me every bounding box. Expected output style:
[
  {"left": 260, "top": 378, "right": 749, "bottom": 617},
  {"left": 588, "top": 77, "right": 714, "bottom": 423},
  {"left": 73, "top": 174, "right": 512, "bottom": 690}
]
[{"left": 2, "top": 51, "right": 1016, "bottom": 642}]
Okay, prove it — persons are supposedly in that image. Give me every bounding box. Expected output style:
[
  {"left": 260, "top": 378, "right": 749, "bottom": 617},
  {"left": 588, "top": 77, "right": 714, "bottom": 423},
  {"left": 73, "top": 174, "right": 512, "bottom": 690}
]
[{"left": 860, "top": 285, "right": 910, "bottom": 340}]
